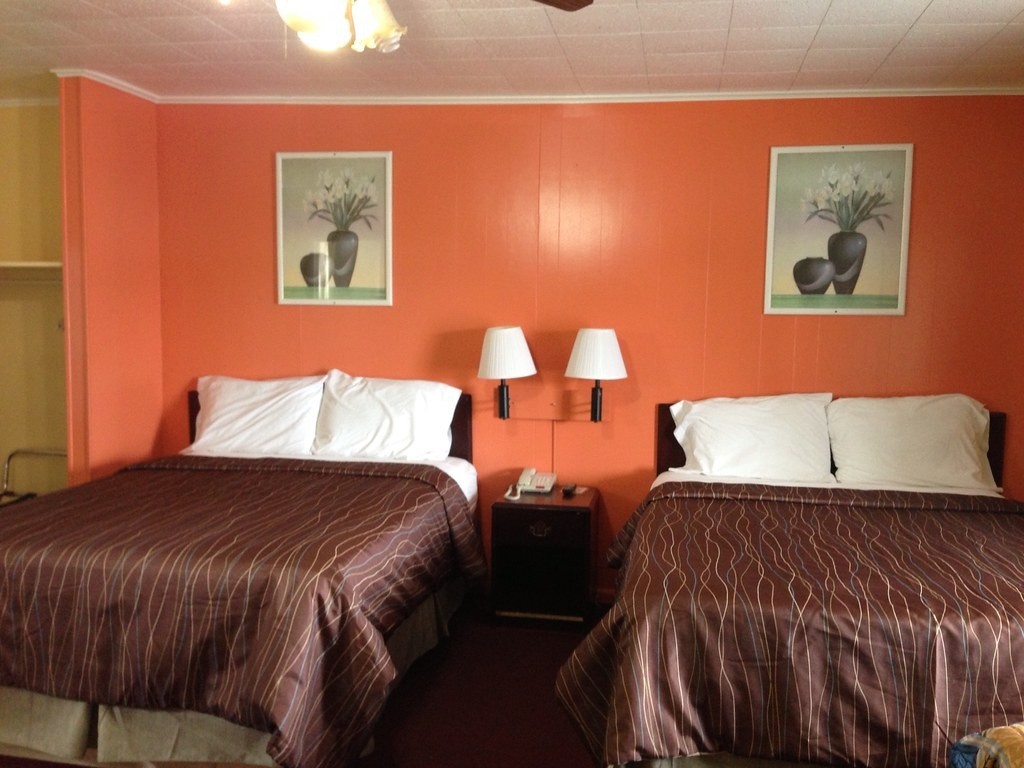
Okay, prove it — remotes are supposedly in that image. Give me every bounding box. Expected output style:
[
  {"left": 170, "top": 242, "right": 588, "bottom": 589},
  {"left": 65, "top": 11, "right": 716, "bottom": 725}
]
[{"left": 563, "top": 483, "right": 577, "bottom": 495}]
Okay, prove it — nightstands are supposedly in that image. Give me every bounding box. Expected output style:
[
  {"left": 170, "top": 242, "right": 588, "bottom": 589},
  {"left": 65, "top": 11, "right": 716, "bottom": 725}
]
[{"left": 490, "top": 485, "right": 597, "bottom": 633}]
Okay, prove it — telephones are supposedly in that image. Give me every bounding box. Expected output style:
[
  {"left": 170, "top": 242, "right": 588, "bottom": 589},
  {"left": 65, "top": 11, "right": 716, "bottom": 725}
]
[{"left": 516, "top": 468, "right": 557, "bottom": 493}]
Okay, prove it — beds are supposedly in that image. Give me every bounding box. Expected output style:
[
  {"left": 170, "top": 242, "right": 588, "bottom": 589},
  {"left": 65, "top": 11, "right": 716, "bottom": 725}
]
[
  {"left": 554, "top": 391, "right": 1024, "bottom": 768},
  {"left": 0, "top": 368, "right": 499, "bottom": 768}
]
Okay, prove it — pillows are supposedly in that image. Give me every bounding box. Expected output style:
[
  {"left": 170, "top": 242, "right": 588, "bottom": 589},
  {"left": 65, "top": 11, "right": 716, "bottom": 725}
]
[
  {"left": 669, "top": 391, "right": 837, "bottom": 484},
  {"left": 827, "top": 394, "right": 1003, "bottom": 492},
  {"left": 178, "top": 373, "right": 328, "bottom": 460},
  {"left": 311, "top": 369, "right": 462, "bottom": 464}
]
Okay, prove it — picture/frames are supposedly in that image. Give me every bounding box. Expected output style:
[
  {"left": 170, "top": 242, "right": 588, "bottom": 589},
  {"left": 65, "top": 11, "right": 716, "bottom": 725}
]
[
  {"left": 275, "top": 152, "right": 392, "bottom": 307},
  {"left": 763, "top": 143, "right": 914, "bottom": 316}
]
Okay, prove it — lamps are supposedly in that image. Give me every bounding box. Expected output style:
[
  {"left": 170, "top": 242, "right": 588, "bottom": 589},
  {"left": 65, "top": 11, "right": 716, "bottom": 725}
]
[
  {"left": 477, "top": 324, "right": 537, "bottom": 419},
  {"left": 563, "top": 327, "right": 628, "bottom": 421},
  {"left": 274, "top": 0, "right": 594, "bottom": 53}
]
